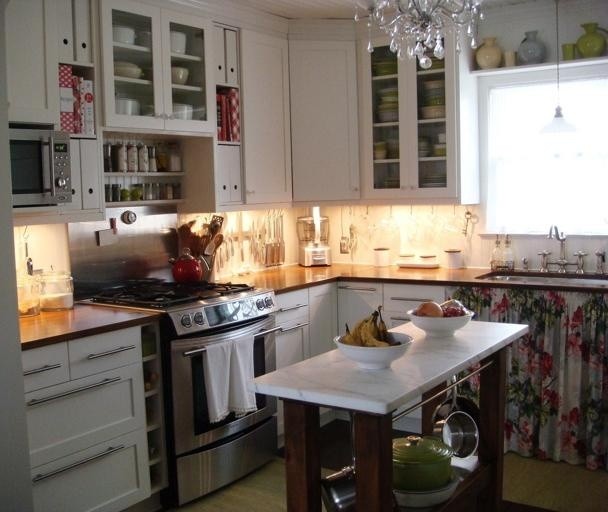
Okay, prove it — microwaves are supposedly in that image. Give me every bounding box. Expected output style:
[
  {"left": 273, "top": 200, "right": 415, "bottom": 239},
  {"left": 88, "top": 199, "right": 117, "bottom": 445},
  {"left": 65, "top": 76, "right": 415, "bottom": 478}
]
[{"left": 8, "top": 129, "right": 71, "bottom": 206}]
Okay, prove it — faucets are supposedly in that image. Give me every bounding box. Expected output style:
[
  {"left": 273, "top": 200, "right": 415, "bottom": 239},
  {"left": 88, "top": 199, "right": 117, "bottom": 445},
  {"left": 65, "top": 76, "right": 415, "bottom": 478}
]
[{"left": 547, "top": 224, "right": 568, "bottom": 274}]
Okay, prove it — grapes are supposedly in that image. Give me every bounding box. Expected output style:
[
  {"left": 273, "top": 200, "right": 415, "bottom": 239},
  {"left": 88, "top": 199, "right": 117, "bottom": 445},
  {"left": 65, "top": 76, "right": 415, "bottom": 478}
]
[{"left": 444, "top": 306, "right": 464, "bottom": 317}]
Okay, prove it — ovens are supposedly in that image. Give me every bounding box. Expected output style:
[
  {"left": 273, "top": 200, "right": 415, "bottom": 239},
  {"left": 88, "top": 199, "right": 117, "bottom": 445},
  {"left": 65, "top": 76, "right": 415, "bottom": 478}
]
[{"left": 162, "top": 316, "right": 277, "bottom": 507}]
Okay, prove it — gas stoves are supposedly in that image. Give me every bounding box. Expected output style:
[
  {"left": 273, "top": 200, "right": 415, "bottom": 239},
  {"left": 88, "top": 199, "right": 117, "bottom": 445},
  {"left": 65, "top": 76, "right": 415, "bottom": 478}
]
[{"left": 92, "top": 278, "right": 277, "bottom": 336}]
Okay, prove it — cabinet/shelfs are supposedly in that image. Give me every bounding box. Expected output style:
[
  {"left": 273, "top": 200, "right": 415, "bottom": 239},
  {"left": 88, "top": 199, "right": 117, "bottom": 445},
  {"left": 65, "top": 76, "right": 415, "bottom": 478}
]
[
  {"left": 288, "top": 18, "right": 362, "bottom": 207},
  {"left": 4, "top": 0, "right": 105, "bottom": 227},
  {"left": 336, "top": 281, "right": 446, "bottom": 435},
  {"left": 177, "top": 0, "right": 294, "bottom": 214},
  {"left": 357, "top": 15, "right": 482, "bottom": 205},
  {"left": 100, "top": 0, "right": 213, "bottom": 207},
  {"left": 22, "top": 321, "right": 170, "bottom": 512},
  {"left": 275, "top": 282, "right": 336, "bottom": 449}
]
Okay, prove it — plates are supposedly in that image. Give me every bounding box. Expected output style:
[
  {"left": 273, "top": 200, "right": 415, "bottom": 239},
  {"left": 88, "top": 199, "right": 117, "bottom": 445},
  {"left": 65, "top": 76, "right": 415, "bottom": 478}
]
[{"left": 372, "top": 52, "right": 446, "bottom": 190}]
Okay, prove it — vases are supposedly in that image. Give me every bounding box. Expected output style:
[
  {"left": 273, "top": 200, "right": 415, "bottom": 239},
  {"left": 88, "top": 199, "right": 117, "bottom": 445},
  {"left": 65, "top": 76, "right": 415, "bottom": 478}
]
[{"left": 476, "top": 23, "right": 608, "bottom": 68}]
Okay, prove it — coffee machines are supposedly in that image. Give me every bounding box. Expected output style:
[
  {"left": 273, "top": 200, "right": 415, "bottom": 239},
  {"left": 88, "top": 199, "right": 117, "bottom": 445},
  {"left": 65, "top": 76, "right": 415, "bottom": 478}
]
[{"left": 298, "top": 215, "right": 331, "bottom": 265}]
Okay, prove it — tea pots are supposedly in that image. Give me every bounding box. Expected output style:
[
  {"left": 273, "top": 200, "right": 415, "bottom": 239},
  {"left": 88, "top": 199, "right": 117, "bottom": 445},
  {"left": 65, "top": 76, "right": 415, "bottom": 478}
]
[
  {"left": 576, "top": 23, "right": 608, "bottom": 59},
  {"left": 170, "top": 248, "right": 206, "bottom": 287}
]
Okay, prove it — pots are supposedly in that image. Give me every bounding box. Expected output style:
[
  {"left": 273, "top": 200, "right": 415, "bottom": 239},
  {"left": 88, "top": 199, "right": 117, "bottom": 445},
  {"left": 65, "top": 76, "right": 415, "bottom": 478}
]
[
  {"left": 393, "top": 435, "right": 451, "bottom": 490},
  {"left": 319, "top": 411, "right": 357, "bottom": 511},
  {"left": 432, "top": 376, "right": 482, "bottom": 460}
]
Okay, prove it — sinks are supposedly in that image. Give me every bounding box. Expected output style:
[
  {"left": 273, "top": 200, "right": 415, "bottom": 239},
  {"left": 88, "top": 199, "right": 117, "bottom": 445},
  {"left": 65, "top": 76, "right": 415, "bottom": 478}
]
[{"left": 474, "top": 270, "right": 608, "bottom": 290}]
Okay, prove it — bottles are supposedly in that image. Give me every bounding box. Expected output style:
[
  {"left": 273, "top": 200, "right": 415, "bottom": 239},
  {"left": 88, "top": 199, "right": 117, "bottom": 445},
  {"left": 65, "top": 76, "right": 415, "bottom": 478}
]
[
  {"left": 371, "top": 247, "right": 461, "bottom": 268},
  {"left": 106, "top": 142, "right": 182, "bottom": 200},
  {"left": 519, "top": 29, "right": 544, "bottom": 63},
  {"left": 503, "top": 241, "right": 514, "bottom": 271},
  {"left": 40, "top": 266, "right": 74, "bottom": 312},
  {"left": 17, "top": 271, "right": 42, "bottom": 317},
  {"left": 476, "top": 37, "right": 501, "bottom": 69},
  {"left": 490, "top": 242, "right": 503, "bottom": 270}
]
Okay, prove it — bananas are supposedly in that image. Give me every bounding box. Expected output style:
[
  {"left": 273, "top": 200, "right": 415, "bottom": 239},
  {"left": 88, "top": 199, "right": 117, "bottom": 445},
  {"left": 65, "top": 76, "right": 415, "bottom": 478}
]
[{"left": 344, "top": 306, "right": 395, "bottom": 347}]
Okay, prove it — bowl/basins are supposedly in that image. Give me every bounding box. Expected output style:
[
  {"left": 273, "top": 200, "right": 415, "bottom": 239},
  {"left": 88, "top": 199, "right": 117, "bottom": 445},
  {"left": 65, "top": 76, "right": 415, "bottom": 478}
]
[
  {"left": 332, "top": 332, "right": 414, "bottom": 371},
  {"left": 392, "top": 480, "right": 457, "bottom": 508},
  {"left": 408, "top": 308, "right": 474, "bottom": 338},
  {"left": 111, "top": 24, "right": 195, "bottom": 121}
]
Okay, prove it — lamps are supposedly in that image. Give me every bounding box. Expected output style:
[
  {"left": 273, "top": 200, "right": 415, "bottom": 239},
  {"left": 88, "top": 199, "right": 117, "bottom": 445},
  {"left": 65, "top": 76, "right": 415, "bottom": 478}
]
[{"left": 354, "top": 0, "right": 485, "bottom": 69}]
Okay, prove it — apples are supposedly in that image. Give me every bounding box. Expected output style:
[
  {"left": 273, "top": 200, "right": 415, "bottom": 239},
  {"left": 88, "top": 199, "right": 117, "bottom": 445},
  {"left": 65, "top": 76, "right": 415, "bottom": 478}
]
[
  {"left": 440, "top": 299, "right": 464, "bottom": 309},
  {"left": 416, "top": 301, "right": 444, "bottom": 317}
]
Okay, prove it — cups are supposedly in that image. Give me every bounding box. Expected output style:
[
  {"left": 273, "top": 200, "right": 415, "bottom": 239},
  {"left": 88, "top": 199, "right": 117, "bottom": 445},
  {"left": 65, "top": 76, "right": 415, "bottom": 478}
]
[
  {"left": 562, "top": 44, "right": 574, "bottom": 61},
  {"left": 504, "top": 51, "right": 516, "bottom": 66}
]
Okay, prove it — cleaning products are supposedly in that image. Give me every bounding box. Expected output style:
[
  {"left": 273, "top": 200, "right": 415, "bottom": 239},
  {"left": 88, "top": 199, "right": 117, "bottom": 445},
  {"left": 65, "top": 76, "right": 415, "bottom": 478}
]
[{"left": 490, "top": 234, "right": 514, "bottom": 272}]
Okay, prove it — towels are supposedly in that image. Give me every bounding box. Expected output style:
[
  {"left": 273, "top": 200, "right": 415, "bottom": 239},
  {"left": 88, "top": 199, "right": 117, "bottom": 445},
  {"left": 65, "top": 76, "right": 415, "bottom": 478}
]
[{"left": 203, "top": 335, "right": 258, "bottom": 423}]
